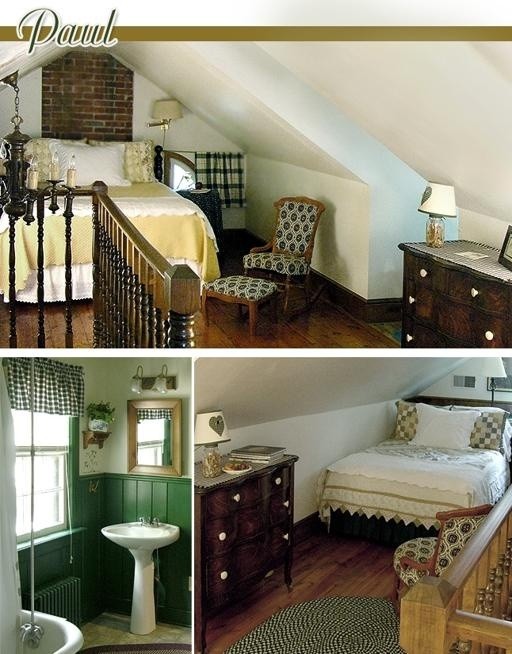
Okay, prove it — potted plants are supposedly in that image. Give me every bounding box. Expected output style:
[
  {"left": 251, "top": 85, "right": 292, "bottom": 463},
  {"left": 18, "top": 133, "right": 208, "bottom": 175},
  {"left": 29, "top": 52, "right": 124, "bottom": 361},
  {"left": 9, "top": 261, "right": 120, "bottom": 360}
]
[{"left": 86, "top": 400, "right": 116, "bottom": 432}]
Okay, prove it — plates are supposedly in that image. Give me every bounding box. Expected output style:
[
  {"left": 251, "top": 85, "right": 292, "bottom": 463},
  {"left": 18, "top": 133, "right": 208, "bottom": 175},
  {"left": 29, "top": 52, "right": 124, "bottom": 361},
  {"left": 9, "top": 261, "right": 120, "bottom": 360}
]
[
  {"left": 191, "top": 189, "right": 211, "bottom": 195},
  {"left": 221, "top": 465, "right": 253, "bottom": 476}
]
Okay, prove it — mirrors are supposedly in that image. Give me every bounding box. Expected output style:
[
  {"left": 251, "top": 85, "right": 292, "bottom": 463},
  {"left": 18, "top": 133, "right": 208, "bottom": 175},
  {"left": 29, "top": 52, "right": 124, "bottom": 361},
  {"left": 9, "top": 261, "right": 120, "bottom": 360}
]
[{"left": 127, "top": 399, "right": 183, "bottom": 478}]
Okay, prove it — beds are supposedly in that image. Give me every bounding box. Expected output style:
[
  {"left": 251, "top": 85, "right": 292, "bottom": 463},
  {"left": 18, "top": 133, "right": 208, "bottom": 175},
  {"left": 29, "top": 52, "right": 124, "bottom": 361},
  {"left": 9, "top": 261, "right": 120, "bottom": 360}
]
[
  {"left": 1, "top": 137, "right": 222, "bottom": 304},
  {"left": 314, "top": 400, "right": 512, "bottom": 551}
]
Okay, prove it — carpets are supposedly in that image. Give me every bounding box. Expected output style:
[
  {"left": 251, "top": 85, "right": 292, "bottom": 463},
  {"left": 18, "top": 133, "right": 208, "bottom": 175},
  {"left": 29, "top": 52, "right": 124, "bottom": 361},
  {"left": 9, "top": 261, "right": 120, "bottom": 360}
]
[
  {"left": 222, "top": 596, "right": 414, "bottom": 654},
  {"left": 75, "top": 643, "right": 191, "bottom": 654}
]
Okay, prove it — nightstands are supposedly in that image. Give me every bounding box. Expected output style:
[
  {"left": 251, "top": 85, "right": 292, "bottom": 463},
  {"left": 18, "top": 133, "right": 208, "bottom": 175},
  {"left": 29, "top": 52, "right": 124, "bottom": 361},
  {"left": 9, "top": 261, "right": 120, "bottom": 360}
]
[{"left": 174, "top": 189, "right": 228, "bottom": 262}]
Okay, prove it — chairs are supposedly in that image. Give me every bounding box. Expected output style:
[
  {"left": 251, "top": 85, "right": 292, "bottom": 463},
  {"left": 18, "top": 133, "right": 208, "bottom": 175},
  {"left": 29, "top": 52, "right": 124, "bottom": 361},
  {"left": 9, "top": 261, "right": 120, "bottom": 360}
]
[
  {"left": 243, "top": 196, "right": 326, "bottom": 323},
  {"left": 392, "top": 504, "right": 493, "bottom": 615}
]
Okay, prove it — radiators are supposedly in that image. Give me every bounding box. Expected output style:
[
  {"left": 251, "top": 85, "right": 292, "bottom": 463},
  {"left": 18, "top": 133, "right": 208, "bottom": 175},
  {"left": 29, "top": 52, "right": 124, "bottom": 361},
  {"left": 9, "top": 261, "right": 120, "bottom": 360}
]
[{"left": 23, "top": 575, "right": 82, "bottom": 631}]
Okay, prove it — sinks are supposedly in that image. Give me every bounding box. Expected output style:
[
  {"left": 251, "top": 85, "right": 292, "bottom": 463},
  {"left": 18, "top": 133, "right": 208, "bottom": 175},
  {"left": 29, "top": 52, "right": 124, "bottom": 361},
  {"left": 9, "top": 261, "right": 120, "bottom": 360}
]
[{"left": 100, "top": 521, "right": 181, "bottom": 549}]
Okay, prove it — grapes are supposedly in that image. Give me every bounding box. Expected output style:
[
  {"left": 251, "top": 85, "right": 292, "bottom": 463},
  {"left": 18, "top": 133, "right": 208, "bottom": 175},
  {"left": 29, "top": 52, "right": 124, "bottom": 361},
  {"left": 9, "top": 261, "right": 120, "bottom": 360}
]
[{"left": 232, "top": 461, "right": 250, "bottom": 471}]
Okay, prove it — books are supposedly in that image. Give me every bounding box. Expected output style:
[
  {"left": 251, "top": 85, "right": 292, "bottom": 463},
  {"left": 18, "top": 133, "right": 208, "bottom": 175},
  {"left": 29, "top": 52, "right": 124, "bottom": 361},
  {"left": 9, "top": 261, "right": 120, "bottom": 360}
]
[
  {"left": 229, "top": 445, "right": 288, "bottom": 464},
  {"left": 190, "top": 187, "right": 211, "bottom": 193}
]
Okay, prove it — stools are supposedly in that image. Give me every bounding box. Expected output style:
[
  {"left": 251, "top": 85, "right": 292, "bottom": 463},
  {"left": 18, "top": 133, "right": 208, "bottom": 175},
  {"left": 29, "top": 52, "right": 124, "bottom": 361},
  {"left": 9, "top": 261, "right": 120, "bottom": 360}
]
[{"left": 203, "top": 275, "right": 278, "bottom": 336}]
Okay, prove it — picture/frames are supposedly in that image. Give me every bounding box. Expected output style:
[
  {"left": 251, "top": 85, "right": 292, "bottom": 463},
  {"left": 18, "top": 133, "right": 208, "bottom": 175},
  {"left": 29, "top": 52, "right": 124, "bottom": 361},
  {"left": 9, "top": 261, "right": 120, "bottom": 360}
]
[
  {"left": 498, "top": 225, "right": 512, "bottom": 272},
  {"left": 486, "top": 358, "right": 512, "bottom": 394}
]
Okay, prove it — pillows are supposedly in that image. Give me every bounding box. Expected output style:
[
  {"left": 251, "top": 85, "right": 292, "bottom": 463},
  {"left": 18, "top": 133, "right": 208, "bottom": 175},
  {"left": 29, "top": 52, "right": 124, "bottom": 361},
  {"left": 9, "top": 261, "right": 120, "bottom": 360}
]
[
  {"left": 449, "top": 406, "right": 511, "bottom": 455},
  {"left": 86, "top": 138, "right": 156, "bottom": 183},
  {"left": 48, "top": 139, "right": 132, "bottom": 187},
  {"left": 388, "top": 400, "right": 453, "bottom": 441},
  {"left": 25, "top": 136, "right": 86, "bottom": 184},
  {"left": 407, "top": 403, "right": 482, "bottom": 450}
]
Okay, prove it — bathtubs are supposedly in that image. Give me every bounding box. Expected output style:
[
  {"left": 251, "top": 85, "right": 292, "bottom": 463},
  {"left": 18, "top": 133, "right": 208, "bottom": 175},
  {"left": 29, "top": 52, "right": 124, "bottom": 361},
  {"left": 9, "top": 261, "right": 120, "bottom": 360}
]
[{"left": 18, "top": 609, "right": 84, "bottom": 653}]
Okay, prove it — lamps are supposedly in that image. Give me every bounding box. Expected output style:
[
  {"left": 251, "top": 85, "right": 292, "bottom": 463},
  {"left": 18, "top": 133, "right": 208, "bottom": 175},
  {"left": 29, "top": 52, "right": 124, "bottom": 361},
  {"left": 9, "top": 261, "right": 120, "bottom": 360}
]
[
  {"left": 194, "top": 409, "right": 231, "bottom": 478},
  {"left": 130, "top": 363, "right": 177, "bottom": 395},
  {"left": 417, "top": 180, "right": 456, "bottom": 248},
  {"left": 0, "top": 68, "right": 77, "bottom": 226},
  {"left": 148, "top": 98, "right": 186, "bottom": 130},
  {"left": 485, "top": 358, "right": 507, "bottom": 406}
]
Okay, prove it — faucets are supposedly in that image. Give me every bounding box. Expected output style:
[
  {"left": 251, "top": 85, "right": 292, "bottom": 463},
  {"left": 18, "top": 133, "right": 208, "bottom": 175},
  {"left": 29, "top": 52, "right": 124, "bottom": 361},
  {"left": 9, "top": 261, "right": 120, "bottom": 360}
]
[{"left": 147, "top": 516, "right": 152, "bottom": 527}]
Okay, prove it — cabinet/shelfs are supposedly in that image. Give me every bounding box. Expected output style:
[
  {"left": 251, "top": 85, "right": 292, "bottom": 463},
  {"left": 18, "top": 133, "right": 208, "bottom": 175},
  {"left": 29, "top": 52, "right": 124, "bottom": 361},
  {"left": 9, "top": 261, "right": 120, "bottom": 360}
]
[
  {"left": 398, "top": 240, "right": 512, "bottom": 348},
  {"left": 194, "top": 450, "right": 299, "bottom": 654}
]
